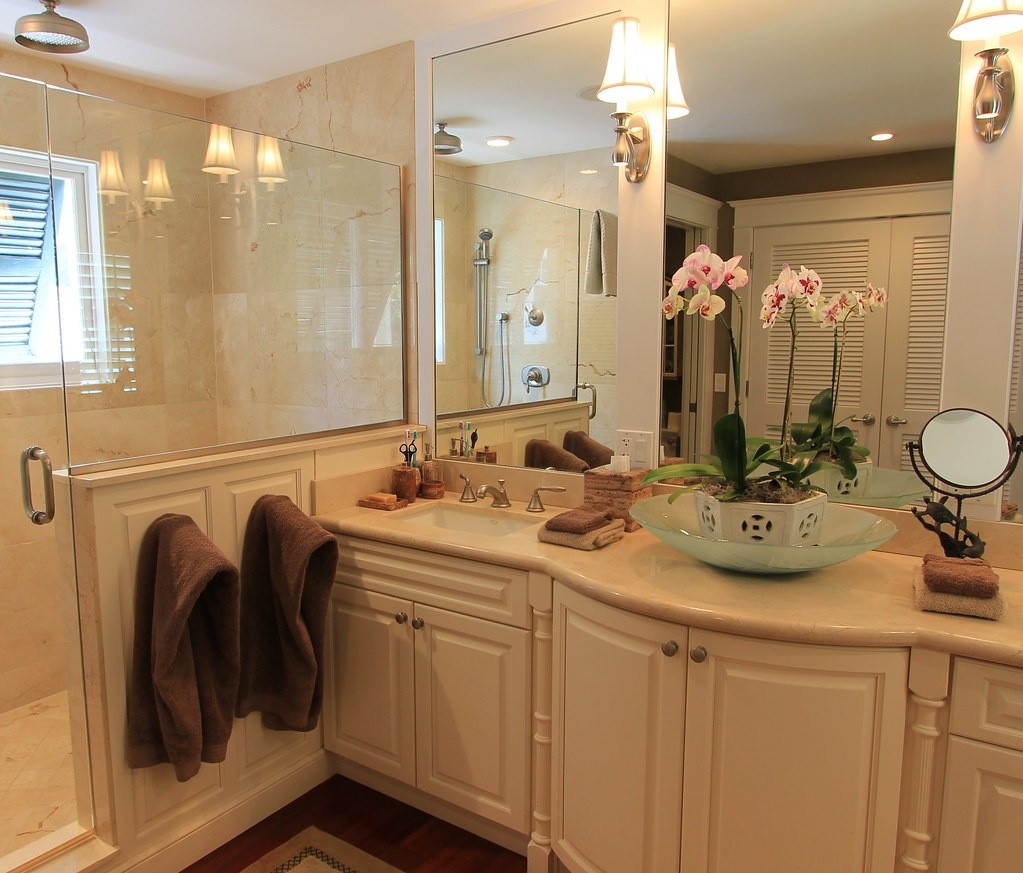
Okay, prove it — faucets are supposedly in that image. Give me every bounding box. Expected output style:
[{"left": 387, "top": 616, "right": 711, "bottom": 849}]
[{"left": 476, "top": 478, "right": 512, "bottom": 508}]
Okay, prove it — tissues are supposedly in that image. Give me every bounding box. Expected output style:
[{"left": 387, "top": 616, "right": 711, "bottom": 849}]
[
  {"left": 583, "top": 456, "right": 653, "bottom": 533},
  {"left": 658, "top": 445, "right": 686, "bottom": 486}
]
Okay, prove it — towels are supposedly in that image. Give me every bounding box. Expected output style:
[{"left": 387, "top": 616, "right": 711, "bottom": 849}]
[
  {"left": 536, "top": 503, "right": 627, "bottom": 551},
  {"left": 562, "top": 429, "right": 615, "bottom": 469},
  {"left": 911, "top": 553, "right": 1009, "bottom": 621},
  {"left": 235, "top": 493, "right": 341, "bottom": 732},
  {"left": 125, "top": 513, "right": 242, "bottom": 783},
  {"left": 525, "top": 438, "right": 590, "bottom": 473},
  {"left": 584, "top": 208, "right": 618, "bottom": 298}
]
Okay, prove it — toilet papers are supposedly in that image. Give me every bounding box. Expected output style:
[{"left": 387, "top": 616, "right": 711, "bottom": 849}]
[{"left": 667, "top": 412, "right": 681, "bottom": 436}]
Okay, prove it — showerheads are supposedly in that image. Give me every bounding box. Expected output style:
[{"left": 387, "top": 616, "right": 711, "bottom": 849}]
[{"left": 478, "top": 227, "right": 493, "bottom": 258}]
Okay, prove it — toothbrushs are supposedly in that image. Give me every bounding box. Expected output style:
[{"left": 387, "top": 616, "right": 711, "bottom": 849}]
[
  {"left": 458, "top": 421, "right": 471, "bottom": 456},
  {"left": 404, "top": 428, "right": 418, "bottom": 468}
]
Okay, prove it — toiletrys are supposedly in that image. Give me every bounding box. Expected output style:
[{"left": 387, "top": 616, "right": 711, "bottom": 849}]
[
  {"left": 392, "top": 461, "right": 417, "bottom": 504},
  {"left": 418, "top": 442, "right": 437, "bottom": 497},
  {"left": 446, "top": 437, "right": 466, "bottom": 462},
  {"left": 475, "top": 445, "right": 497, "bottom": 464}
]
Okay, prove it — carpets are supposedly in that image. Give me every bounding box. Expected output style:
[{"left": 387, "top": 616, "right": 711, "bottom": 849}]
[{"left": 237, "top": 826, "right": 408, "bottom": 873}]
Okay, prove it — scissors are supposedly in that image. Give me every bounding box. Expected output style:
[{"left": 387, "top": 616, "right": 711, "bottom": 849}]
[
  {"left": 470, "top": 428, "right": 478, "bottom": 449},
  {"left": 399, "top": 438, "right": 417, "bottom": 467}
]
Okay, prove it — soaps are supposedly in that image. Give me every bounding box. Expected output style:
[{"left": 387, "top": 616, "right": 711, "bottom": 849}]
[{"left": 366, "top": 492, "right": 397, "bottom": 503}]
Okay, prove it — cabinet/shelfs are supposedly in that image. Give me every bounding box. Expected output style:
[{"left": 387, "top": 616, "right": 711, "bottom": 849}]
[
  {"left": 71, "top": 450, "right": 325, "bottom": 866},
  {"left": 934, "top": 653, "right": 1023, "bottom": 873},
  {"left": 323, "top": 532, "right": 531, "bottom": 860},
  {"left": 661, "top": 283, "right": 683, "bottom": 381},
  {"left": 503, "top": 404, "right": 590, "bottom": 469},
  {"left": 552, "top": 576, "right": 910, "bottom": 873}
]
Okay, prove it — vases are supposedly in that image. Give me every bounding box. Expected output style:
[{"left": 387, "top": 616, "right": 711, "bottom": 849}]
[
  {"left": 784, "top": 456, "right": 872, "bottom": 499},
  {"left": 695, "top": 490, "right": 828, "bottom": 549}
]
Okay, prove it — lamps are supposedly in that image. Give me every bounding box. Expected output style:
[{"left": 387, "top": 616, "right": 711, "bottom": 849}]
[
  {"left": 485, "top": 136, "right": 510, "bottom": 149},
  {"left": 596, "top": 16, "right": 654, "bottom": 184},
  {"left": 13, "top": 1, "right": 90, "bottom": 55},
  {"left": 96, "top": 148, "right": 174, "bottom": 237},
  {"left": 945, "top": 1, "right": 1023, "bottom": 145},
  {"left": 667, "top": 42, "right": 690, "bottom": 123},
  {"left": 433, "top": 121, "right": 463, "bottom": 156},
  {"left": 203, "top": 123, "right": 286, "bottom": 232}
]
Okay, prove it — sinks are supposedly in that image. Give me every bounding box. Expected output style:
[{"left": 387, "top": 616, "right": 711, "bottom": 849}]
[{"left": 394, "top": 506, "right": 541, "bottom": 537}]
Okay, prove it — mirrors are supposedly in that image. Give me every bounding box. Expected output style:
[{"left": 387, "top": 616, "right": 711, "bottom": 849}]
[
  {"left": 656, "top": 0, "right": 963, "bottom": 515},
  {"left": 431, "top": 8, "right": 624, "bottom": 472},
  {"left": 905, "top": 407, "right": 1023, "bottom": 559},
  {"left": 999, "top": 233, "right": 1022, "bottom": 523},
  {"left": 45, "top": 74, "right": 408, "bottom": 468}
]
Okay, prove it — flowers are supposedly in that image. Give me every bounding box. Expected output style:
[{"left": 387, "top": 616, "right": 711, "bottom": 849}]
[
  {"left": 640, "top": 245, "right": 833, "bottom": 503},
  {"left": 766, "top": 283, "right": 888, "bottom": 482}
]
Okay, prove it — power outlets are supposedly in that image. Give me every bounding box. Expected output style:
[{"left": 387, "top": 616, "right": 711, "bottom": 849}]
[{"left": 615, "top": 429, "right": 653, "bottom": 470}]
